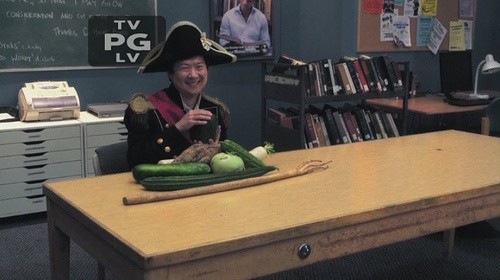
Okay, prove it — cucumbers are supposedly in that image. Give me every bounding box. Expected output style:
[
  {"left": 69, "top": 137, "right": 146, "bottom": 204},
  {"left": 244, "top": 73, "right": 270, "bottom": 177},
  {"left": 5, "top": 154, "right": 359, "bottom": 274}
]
[{"left": 131, "top": 162, "right": 211, "bottom": 181}]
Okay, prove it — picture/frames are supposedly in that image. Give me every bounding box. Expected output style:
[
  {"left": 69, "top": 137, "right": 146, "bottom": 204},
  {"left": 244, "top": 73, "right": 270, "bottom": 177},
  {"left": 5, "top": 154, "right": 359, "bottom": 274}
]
[{"left": 209, "top": 0, "right": 274, "bottom": 62}]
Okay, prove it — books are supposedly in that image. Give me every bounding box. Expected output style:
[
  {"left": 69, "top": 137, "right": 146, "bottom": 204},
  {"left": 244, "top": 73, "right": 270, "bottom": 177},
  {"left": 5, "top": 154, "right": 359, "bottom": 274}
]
[
  {"left": 267, "top": 104, "right": 399, "bottom": 149},
  {"left": 271, "top": 54, "right": 405, "bottom": 98},
  {"left": 223, "top": 0, "right": 264, "bottom": 15}
]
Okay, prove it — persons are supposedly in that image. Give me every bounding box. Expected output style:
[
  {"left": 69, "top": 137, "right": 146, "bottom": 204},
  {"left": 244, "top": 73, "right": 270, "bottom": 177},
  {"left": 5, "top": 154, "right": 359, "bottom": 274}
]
[
  {"left": 219, "top": 0, "right": 271, "bottom": 52},
  {"left": 124, "top": 21, "right": 236, "bottom": 172}
]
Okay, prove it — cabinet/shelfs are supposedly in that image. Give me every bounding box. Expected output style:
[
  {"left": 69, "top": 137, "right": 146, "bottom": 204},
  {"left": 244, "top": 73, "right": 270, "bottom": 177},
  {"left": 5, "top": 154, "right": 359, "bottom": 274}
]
[
  {"left": 259, "top": 62, "right": 408, "bottom": 153},
  {"left": 0, "top": 111, "right": 129, "bottom": 218}
]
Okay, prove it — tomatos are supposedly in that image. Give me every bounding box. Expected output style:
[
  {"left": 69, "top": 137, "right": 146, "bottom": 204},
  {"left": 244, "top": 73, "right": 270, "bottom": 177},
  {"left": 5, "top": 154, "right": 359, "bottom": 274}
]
[{"left": 210, "top": 151, "right": 245, "bottom": 173}]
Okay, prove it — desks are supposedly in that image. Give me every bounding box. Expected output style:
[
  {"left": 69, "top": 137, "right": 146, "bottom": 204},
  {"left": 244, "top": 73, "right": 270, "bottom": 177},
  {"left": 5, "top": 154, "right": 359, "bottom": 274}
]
[
  {"left": 365, "top": 90, "right": 500, "bottom": 135},
  {"left": 42, "top": 129, "right": 500, "bottom": 280}
]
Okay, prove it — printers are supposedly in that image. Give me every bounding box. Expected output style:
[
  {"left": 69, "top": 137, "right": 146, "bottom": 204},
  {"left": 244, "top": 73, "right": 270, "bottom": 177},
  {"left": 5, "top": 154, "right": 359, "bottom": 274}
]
[{"left": 17, "top": 81, "right": 80, "bottom": 122}]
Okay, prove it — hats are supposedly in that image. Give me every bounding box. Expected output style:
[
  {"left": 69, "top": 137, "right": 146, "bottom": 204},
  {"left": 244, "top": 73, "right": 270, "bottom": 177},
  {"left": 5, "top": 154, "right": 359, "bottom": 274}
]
[{"left": 136, "top": 20, "right": 238, "bottom": 74}]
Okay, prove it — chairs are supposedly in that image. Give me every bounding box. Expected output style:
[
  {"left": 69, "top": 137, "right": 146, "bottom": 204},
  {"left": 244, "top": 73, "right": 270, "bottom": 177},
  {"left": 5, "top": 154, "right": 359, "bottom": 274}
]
[{"left": 92, "top": 142, "right": 130, "bottom": 176}]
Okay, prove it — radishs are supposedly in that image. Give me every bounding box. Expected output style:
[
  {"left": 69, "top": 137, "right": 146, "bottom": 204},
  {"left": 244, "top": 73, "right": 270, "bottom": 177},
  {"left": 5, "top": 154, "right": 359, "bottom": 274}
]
[{"left": 248, "top": 141, "right": 276, "bottom": 160}]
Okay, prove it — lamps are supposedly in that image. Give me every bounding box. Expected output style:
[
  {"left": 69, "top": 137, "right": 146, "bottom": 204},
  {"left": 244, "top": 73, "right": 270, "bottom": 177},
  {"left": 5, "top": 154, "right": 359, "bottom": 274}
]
[{"left": 464, "top": 54, "right": 500, "bottom": 99}]
